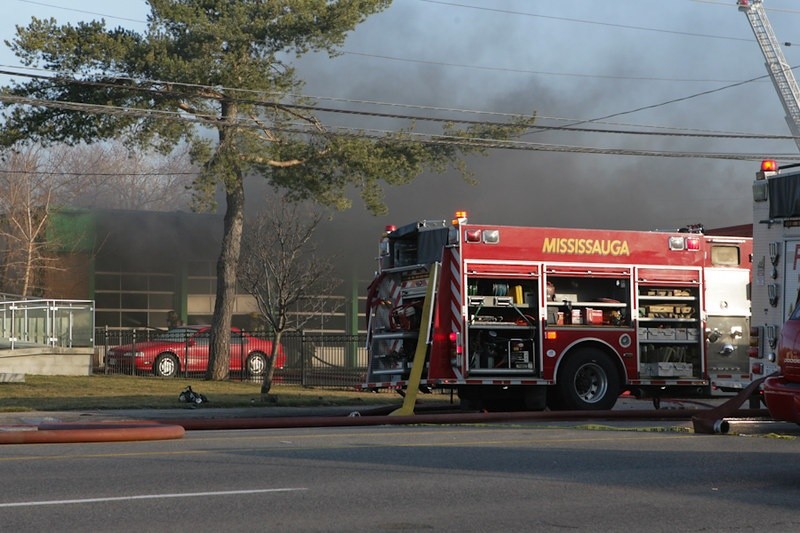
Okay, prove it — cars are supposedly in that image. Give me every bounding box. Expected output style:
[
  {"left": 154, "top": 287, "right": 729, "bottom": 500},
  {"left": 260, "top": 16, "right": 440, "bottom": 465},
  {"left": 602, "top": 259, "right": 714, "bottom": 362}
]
[{"left": 103, "top": 325, "right": 287, "bottom": 379}]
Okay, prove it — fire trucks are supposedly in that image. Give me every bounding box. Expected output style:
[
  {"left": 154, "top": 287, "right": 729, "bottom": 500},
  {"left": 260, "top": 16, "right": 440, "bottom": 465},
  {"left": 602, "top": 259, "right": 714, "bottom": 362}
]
[
  {"left": 360, "top": 211, "right": 755, "bottom": 418},
  {"left": 736, "top": 0, "right": 800, "bottom": 427}
]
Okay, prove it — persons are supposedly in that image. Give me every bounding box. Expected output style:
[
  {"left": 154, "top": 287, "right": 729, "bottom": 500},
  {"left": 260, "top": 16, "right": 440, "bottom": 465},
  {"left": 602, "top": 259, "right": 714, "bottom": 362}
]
[
  {"left": 304, "top": 337, "right": 315, "bottom": 369},
  {"left": 167, "top": 311, "right": 178, "bottom": 330},
  {"left": 391, "top": 306, "right": 431, "bottom": 397}
]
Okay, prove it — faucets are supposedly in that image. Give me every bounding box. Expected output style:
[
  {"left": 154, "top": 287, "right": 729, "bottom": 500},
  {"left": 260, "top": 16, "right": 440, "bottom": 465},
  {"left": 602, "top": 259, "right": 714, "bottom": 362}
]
[
  {"left": 708, "top": 330, "right": 722, "bottom": 343},
  {"left": 729, "top": 330, "right": 742, "bottom": 339}
]
